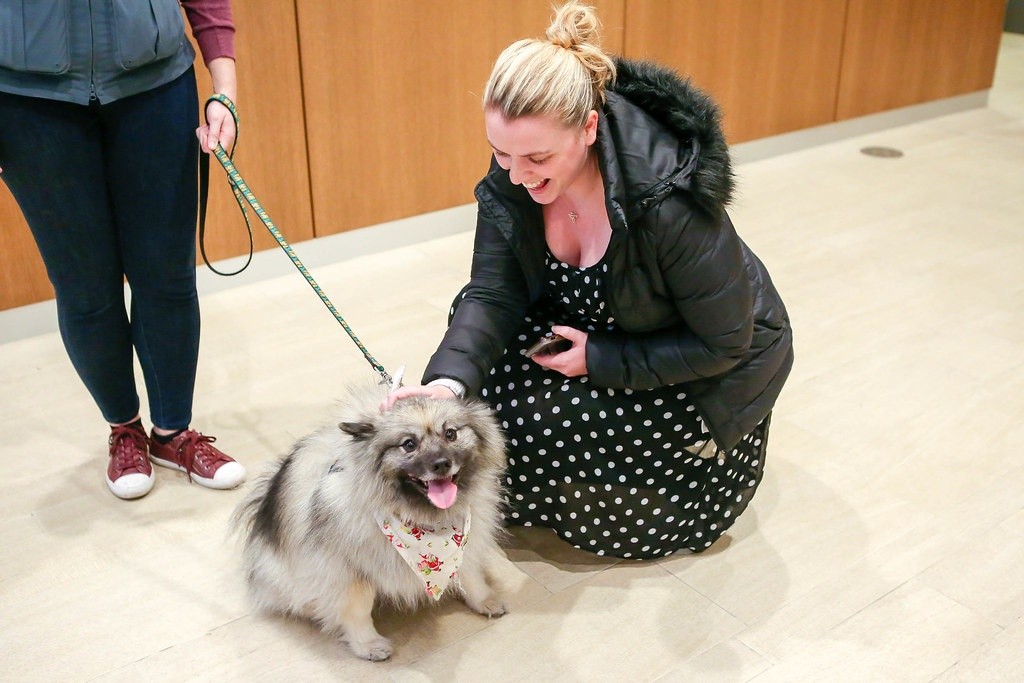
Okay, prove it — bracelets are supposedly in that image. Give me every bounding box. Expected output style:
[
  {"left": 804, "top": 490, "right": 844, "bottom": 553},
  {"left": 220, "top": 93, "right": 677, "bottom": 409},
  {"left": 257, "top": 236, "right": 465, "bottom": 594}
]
[{"left": 425, "top": 378, "right": 464, "bottom": 402}]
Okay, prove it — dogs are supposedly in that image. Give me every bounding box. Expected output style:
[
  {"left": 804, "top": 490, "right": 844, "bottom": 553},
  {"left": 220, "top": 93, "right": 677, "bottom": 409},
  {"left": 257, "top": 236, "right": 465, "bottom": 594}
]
[{"left": 225, "top": 367, "right": 528, "bottom": 660}]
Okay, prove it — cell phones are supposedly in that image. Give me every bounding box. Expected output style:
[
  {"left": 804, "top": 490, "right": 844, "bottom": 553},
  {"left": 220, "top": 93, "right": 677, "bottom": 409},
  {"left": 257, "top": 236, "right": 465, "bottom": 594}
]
[{"left": 525, "top": 335, "right": 566, "bottom": 358}]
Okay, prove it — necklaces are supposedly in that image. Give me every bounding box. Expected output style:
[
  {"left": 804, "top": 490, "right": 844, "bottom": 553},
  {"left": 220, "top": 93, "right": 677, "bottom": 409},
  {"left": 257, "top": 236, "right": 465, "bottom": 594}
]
[{"left": 567, "top": 207, "right": 583, "bottom": 223}]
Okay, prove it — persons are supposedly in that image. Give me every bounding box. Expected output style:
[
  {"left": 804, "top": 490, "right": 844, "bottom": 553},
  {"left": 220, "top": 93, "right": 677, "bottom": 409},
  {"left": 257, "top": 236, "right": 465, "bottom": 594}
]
[
  {"left": 379, "top": 0, "right": 794, "bottom": 561},
  {"left": 0, "top": 0, "right": 248, "bottom": 501}
]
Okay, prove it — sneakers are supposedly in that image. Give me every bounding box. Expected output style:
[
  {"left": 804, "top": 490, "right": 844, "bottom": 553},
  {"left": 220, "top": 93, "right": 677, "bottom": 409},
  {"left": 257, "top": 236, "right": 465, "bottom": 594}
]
[
  {"left": 106, "top": 424, "right": 155, "bottom": 500},
  {"left": 148, "top": 426, "right": 247, "bottom": 490}
]
